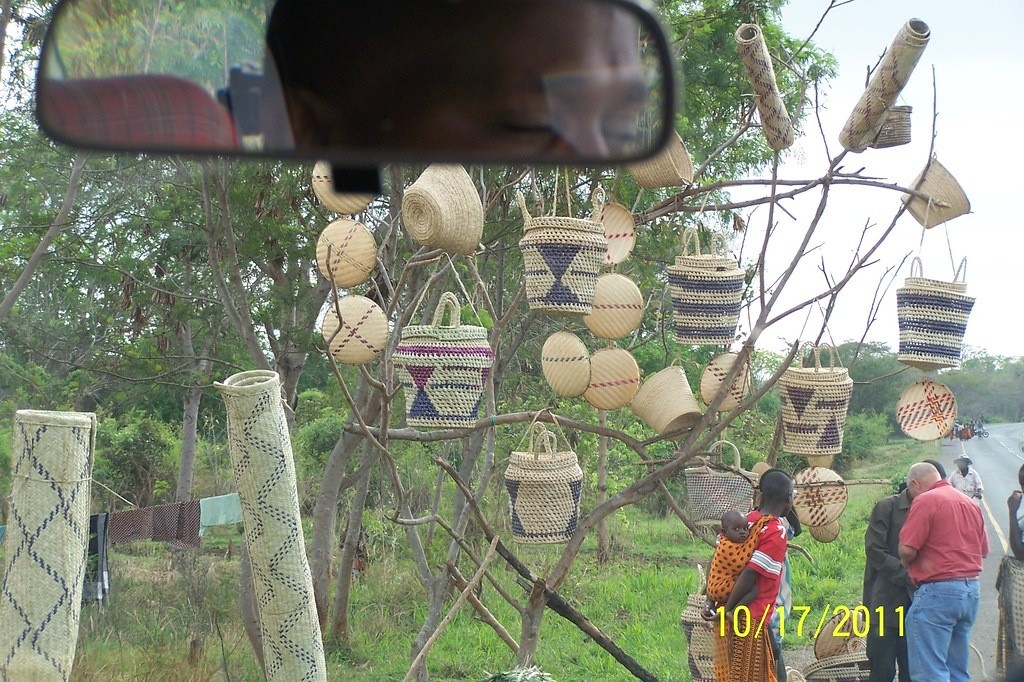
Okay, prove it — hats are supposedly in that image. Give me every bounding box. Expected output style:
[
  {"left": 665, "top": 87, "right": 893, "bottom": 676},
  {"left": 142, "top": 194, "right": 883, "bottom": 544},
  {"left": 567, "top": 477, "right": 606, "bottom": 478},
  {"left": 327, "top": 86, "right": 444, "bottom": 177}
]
[{"left": 953, "top": 454, "right": 973, "bottom": 465}]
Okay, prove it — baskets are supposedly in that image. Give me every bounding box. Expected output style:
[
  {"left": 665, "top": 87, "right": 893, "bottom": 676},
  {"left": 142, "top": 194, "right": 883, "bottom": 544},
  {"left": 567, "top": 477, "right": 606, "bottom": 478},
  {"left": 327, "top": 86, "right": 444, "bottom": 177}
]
[
  {"left": 631, "top": 356, "right": 703, "bottom": 442},
  {"left": 628, "top": 127, "right": 696, "bottom": 190},
  {"left": 897, "top": 255, "right": 977, "bottom": 372},
  {"left": 512, "top": 164, "right": 645, "bottom": 341},
  {"left": 901, "top": 152, "right": 974, "bottom": 230},
  {"left": 402, "top": 162, "right": 484, "bottom": 258},
  {"left": 541, "top": 330, "right": 642, "bottom": 411},
  {"left": 790, "top": 465, "right": 850, "bottom": 544},
  {"left": 697, "top": 351, "right": 754, "bottom": 413},
  {"left": 311, "top": 160, "right": 379, "bottom": 216},
  {"left": 785, "top": 609, "right": 871, "bottom": 682},
  {"left": 501, "top": 408, "right": 585, "bottom": 545},
  {"left": 777, "top": 297, "right": 854, "bottom": 456},
  {"left": 895, "top": 380, "right": 959, "bottom": 444},
  {"left": 393, "top": 290, "right": 496, "bottom": 431},
  {"left": 320, "top": 292, "right": 390, "bottom": 366},
  {"left": 315, "top": 217, "right": 378, "bottom": 291},
  {"left": 666, "top": 225, "right": 745, "bottom": 346},
  {"left": 869, "top": 92, "right": 913, "bottom": 150},
  {"left": 679, "top": 563, "right": 719, "bottom": 681},
  {"left": 684, "top": 441, "right": 759, "bottom": 528}
]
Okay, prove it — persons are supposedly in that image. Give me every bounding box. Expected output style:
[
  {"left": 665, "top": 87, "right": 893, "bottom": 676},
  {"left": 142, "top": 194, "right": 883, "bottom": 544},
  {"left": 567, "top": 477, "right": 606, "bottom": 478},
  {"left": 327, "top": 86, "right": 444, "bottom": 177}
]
[
  {"left": 948, "top": 454, "right": 984, "bottom": 503},
  {"left": 1006, "top": 464, "right": 1024, "bottom": 560},
  {"left": 707, "top": 510, "right": 771, "bottom": 601},
  {"left": 862, "top": 460, "right": 946, "bottom": 682},
  {"left": 944, "top": 413, "right": 994, "bottom": 440},
  {"left": 896, "top": 463, "right": 988, "bottom": 682},
  {"left": 263, "top": 0, "right": 647, "bottom": 159},
  {"left": 700, "top": 469, "right": 794, "bottom": 682}
]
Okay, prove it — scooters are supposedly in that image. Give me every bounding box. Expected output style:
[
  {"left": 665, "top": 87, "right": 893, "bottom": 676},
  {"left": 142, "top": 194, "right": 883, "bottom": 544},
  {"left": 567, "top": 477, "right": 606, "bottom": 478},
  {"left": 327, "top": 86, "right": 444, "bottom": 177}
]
[{"left": 972, "top": 428, "right": 989, "bottom": 438}]
